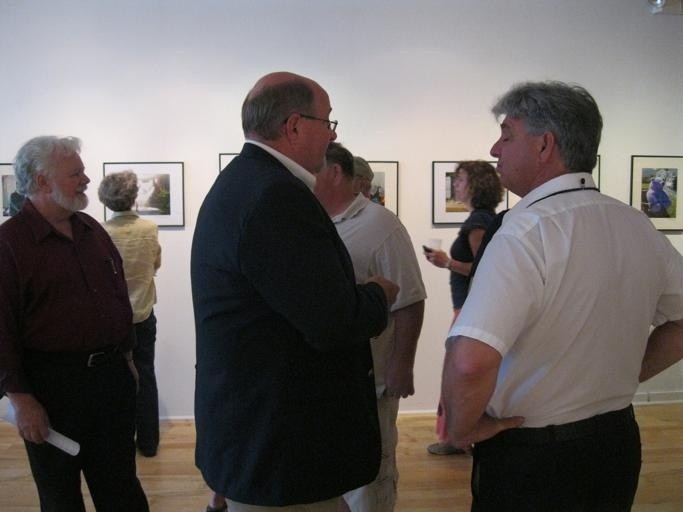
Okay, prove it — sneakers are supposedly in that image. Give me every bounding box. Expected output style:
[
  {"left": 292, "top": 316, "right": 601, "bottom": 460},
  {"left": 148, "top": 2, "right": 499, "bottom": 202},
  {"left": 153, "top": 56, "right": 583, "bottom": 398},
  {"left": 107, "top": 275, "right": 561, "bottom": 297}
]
[{"left": 427, "top": 443, "right": 466, "bottom": 455}]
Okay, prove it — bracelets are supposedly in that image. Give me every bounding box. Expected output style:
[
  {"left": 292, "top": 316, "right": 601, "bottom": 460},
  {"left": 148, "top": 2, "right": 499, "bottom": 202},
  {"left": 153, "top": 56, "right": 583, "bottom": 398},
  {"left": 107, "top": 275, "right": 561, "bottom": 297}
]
[
  {"left": 446, "top": 257, "right": 455, "bottom": 272},
  {"left": 125, "top": 358, "right": 134, "bottom": 362}
]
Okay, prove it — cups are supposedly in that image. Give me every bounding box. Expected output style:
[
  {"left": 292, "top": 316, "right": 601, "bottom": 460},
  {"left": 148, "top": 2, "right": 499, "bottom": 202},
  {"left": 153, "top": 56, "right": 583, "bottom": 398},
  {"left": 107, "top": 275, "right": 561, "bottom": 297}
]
[{"left": 426, "top": 237, "right": 442, "bottom": 250}]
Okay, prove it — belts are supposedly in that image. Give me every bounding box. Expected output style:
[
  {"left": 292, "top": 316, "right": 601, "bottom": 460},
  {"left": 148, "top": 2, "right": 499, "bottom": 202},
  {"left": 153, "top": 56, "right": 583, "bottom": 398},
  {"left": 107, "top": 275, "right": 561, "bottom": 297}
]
[
  {"left": 502, "top": 405, "right": 634, "bottom": 445},
  {"left": 50, "top": 351, "right": 106, "bottom": 367}
]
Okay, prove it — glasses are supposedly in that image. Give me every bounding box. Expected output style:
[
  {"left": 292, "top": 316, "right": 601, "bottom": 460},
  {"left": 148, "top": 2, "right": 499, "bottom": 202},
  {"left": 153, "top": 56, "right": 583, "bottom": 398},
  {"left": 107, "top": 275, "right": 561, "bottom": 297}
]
[{"left": 284, "top": 113, "right": 338, "bottom": 132}]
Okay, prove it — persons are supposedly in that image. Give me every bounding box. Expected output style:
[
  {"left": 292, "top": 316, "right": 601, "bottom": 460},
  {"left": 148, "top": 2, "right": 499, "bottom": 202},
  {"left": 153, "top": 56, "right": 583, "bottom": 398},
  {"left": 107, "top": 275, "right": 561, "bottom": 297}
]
[
  {"left": 190, "top": 70, "right": 398, "bottom": 510},
  {"left": 421, "top": 160, "right": 503, "bottom": 454},
  {"left": 315, "top": 143, "right": 430, "bottom": 512},
  {"left": 352, "top": 157, "right": 374, "bottom": 200},
  {"left": 441, "top": 80, "right": 683, "bottom": 510},
  {"left": 96, "top": 171, "right": 163, "bottom": 459},
  {"left": 0, "top": 136, "right": 149, "bottom": 511}
]
